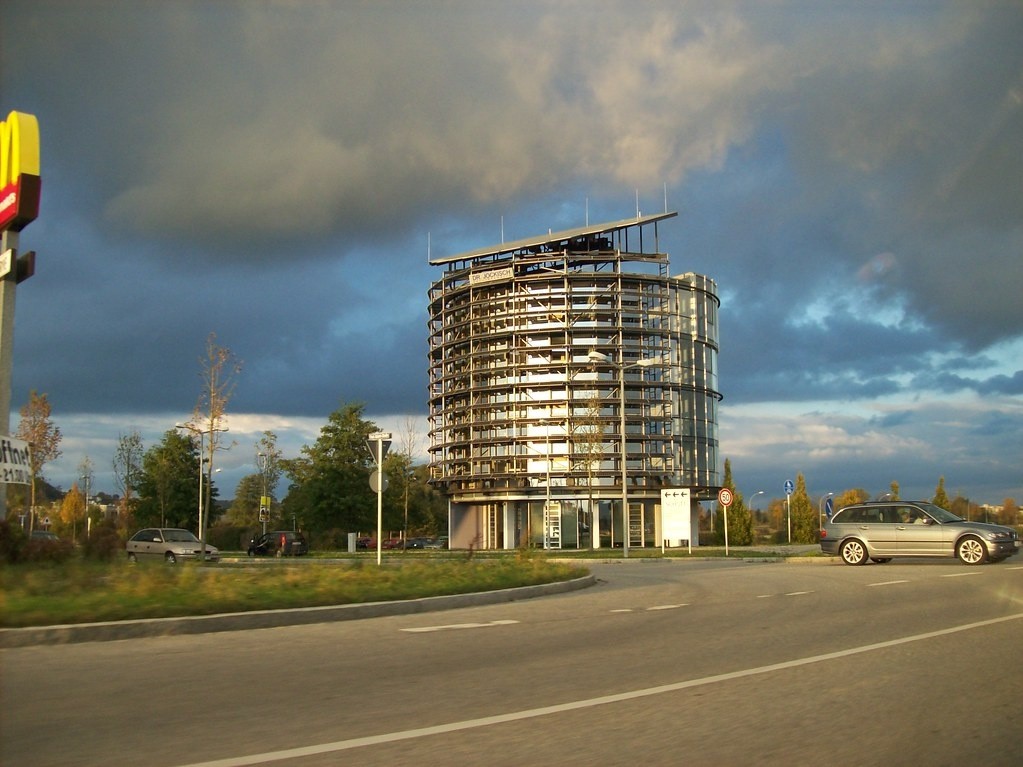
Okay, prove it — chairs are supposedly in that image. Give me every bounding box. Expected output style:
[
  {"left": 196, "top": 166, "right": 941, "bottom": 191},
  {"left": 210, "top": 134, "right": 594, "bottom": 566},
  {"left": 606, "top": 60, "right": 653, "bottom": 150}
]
[{"left": 879, "top": 512, "right": 883, "bottom": 522}]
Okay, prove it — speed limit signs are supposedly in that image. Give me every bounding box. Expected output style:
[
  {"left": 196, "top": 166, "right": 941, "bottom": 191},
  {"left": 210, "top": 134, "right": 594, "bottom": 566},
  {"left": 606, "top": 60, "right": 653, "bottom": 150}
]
[{"left": 718, "top": 488, "right": 733, "bottom": 506}]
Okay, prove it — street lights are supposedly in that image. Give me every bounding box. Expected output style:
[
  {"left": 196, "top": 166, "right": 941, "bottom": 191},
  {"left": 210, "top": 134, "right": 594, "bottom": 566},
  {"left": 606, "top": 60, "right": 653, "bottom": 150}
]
[
  {"left": 748, "top": 491, "right": 764, "bottom": 517},
  {"left": 175, "top": 422, "right": 230, "bottom": 542},
  {"left": 540, "top": 419, "right": 552, "bottom": 553},
  {"left": 258, "top": 452, "right": 278, "bottom": 540},
  {"left": 819, "top": 493, "right": 835, "bottom": 532},
  {"left": 80, "top": 474, "right": 90, "bottom": 523},
  {"left": 587, "top": 350, "right": 663, "bottom": 560}
]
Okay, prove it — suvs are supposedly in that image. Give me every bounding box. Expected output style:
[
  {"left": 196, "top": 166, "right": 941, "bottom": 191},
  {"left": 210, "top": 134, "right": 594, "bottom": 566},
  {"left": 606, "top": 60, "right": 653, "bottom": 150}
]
[{"left": 818, "top": 500, "right": 1023, "bottom": 567}]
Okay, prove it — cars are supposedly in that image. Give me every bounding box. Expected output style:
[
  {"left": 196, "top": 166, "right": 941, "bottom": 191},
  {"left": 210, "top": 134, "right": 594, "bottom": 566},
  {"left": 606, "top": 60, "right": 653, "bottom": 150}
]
[
  {"left": 247, "top": 531, "right": 308, "bottom": 558},
  {"left": 357, "top": 537, "right": 375, "bottom": 551},
  {"left": 398, "top": 535, "right": 448, "bottom": 550},
  {"left": 125, "top": 529, "right": 221, "bottom": 566},
  {"left": 381, "top": 539, "right": 398, "bottom": 551}
]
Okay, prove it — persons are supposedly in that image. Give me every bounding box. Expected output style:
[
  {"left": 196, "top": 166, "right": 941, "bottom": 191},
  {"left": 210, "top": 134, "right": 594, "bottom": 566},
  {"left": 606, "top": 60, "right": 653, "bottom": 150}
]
[{"left": 914, "top": 511, "right": 930, "bottom": 524}]
